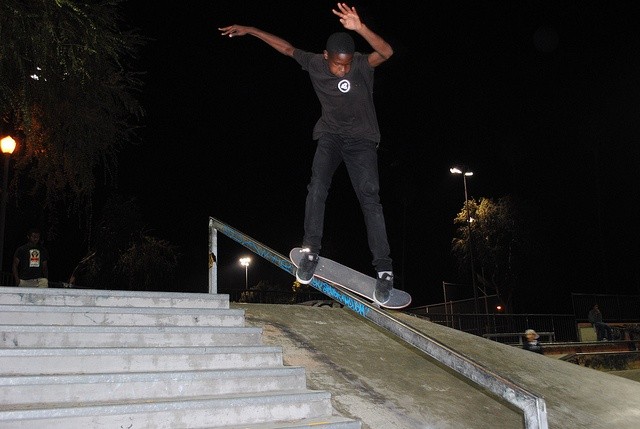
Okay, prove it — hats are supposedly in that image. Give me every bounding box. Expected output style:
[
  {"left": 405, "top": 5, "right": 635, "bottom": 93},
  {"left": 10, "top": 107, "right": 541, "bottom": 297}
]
[{"left": 524, "top": 329, "right": 540, "bottom": 340}]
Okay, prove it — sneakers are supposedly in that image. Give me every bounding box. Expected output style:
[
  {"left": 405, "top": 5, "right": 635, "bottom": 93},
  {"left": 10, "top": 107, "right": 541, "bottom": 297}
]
[
  {"left": 373, "top": 273, "right": 394, "bottom": 304},
  {"left": 296, "top": 253, "right": 318, "bottom": 284}
]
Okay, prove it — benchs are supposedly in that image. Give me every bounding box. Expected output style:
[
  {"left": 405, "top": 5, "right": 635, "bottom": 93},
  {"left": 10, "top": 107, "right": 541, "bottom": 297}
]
[{"left": 484, "top": 332, "right": 557, "bottom": 344}]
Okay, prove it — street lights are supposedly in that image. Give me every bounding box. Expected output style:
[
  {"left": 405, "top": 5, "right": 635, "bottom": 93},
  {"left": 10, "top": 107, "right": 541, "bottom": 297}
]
[
  {"left": 239, "top": 257, "right": 251, "bottom": 289},
  {"left": 0, "top": 133, "right": 17, "bottom": 286},
  {"left": 449, "top": 166, "right": 483, "bottom": 336}
]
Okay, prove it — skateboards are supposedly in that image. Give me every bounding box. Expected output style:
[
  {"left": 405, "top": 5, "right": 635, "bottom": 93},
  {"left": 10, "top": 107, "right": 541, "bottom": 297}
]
[{"left": 289, "top": 247, "right": 412, "bottom": 309}]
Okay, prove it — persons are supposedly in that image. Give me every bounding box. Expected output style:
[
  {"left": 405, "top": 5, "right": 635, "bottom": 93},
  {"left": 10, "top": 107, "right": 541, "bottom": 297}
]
[
  {"left": 219, "top": 3, "right": 395, "bottom": 306},
  {"left": 588, "top": 303, "right": 612, "bottom": 342},
  {"left": 521, "top": 329, "right": 543, "bottom": 355},
  {"left": 11, "top": 229, "right": 50, "bottom": 289}
]
